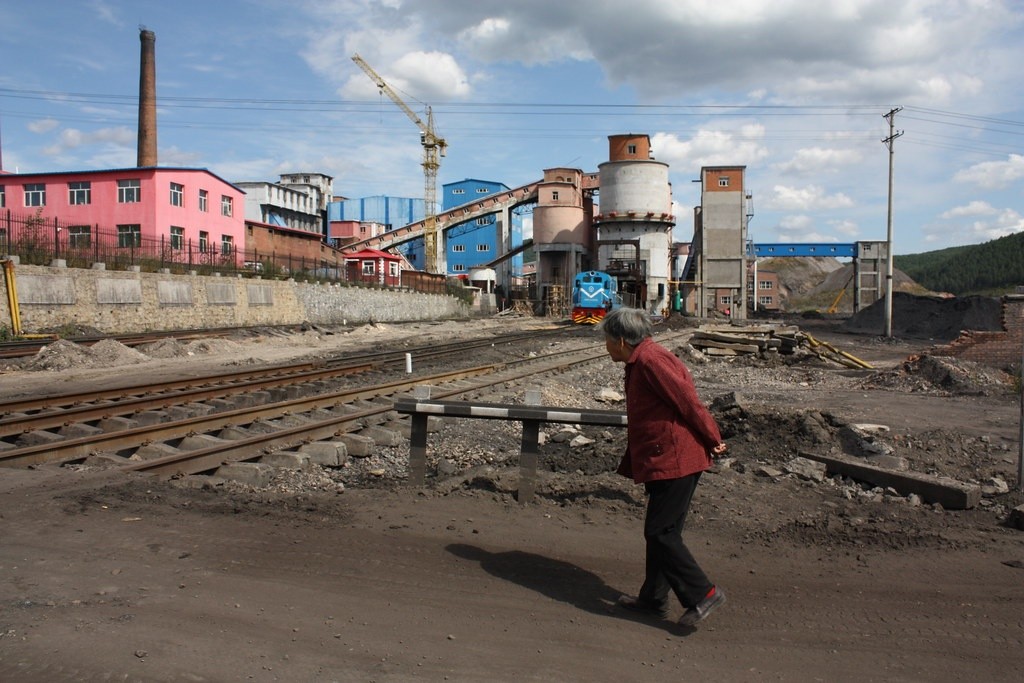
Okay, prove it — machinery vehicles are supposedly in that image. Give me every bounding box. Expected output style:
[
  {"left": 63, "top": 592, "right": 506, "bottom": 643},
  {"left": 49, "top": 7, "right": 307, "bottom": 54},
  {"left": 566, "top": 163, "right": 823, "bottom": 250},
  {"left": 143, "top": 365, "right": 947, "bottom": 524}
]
[{"left": 817, "top": 272, "right": 854, "bottom": 314}]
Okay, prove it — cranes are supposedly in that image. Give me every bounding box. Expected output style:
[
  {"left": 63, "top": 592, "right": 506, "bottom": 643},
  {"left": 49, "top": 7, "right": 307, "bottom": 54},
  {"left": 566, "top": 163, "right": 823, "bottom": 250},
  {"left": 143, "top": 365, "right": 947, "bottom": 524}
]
[{"left": 351, "top": 52, "right": 449, "bottom": 281}]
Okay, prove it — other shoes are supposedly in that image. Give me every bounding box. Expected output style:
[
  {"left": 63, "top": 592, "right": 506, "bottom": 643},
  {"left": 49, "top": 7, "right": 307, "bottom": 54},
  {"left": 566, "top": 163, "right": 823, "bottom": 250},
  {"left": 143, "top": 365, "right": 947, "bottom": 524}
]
[
  {"left": 619, "top": 591, "right": 672, "bottom": 617},
  {"left": 678, "top": 585, "right": 727, "bottom": 628}
]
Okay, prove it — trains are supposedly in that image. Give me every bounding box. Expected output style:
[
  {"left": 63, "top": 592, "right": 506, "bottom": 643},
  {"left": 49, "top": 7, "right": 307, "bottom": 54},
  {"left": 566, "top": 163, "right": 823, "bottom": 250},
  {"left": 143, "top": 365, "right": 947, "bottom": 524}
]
[{"left": 572, "top": 270, "right": 623, "bottom": 325}]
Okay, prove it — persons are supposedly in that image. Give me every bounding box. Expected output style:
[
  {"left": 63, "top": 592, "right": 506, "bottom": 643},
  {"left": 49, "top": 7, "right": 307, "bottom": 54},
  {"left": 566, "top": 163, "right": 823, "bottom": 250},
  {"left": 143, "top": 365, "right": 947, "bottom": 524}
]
[{"left": 595, "top": 307, "right": 727, "bottom": 628}]
